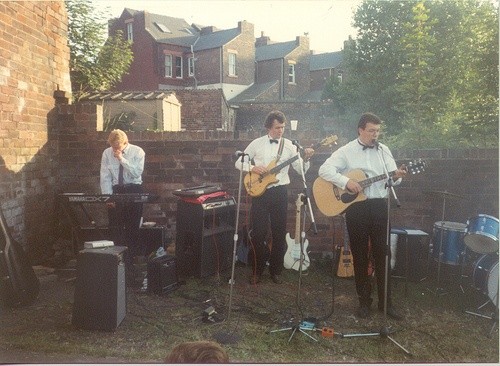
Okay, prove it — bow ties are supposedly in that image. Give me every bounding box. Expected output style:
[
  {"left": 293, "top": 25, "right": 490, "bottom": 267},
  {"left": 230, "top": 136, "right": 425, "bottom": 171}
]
[
  {"left": 363, "top": 146, "right": 374, "bottom": 150},
  {"left": 270, "top": 139, "right": 279, "bottom": 144}
]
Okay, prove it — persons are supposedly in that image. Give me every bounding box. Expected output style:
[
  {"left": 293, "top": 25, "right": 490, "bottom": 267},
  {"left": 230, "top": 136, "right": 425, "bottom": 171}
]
[
  {"left": 99, "top": 128, "right": 145, "bottom": 248},
  {"left": 318, "top": 112, "right": 408, "bottom": 322},
  {"left": 163, "top": 340, "right": 229, "bottom": 364},
  {"left": 234, "top": 109, "right": 316, "bottom": 286}
]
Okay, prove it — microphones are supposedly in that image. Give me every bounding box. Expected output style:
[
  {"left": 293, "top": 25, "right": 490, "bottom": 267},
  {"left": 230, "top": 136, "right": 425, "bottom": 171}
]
[
  {"left": 292, "top": 140, "right": 303, "bottom": 151},
  {"left": 235, "top": 150, "right": 249, "bottom": 157},
  {"left": 371, "top": 139, "right": 382, "bottom": 148}
]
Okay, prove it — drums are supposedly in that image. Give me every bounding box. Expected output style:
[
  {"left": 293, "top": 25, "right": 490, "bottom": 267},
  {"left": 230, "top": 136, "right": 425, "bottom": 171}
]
[
  {"left": 462, "top": 213, "right": 499, "bottom": 253},
  {"left": 472, "top": 249, "right": 499, "bottom": 307},
  {"left": 431, "top": 221, "right": 471, "bottom": 267}
]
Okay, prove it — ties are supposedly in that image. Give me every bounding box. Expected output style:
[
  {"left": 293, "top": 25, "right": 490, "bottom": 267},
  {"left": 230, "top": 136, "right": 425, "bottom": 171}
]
[{"left": 118, "top": 152, "right": 124, "bottom": 186}]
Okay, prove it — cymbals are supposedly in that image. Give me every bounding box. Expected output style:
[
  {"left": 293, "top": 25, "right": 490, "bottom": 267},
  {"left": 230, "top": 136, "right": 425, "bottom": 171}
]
[{"left": 422, "top": 189, "right": 468, "bottom": 201}]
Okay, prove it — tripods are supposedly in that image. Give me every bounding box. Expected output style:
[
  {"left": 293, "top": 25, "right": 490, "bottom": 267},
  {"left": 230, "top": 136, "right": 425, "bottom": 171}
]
[{"left": 342, "top": 145, "right": 414, "bottom": 357}]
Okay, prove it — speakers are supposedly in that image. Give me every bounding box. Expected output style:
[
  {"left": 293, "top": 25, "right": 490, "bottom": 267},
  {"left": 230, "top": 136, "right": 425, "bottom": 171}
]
[
  {"left": 73, "top": 245, "right": 129, "bottom": 331},
  {"left": 176, "top": 198, "right": 237, "bottom": 279},
  {"left": 390, "top": 227, "right": 428, "bottom": 282}
]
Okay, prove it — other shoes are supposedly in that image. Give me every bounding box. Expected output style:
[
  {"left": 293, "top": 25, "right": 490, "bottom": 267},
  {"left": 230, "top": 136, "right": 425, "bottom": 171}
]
[
  {"left": 359, "top": 303, "right": 371, "bottom": 317},
  {"left": 271, "top": 273, "right": 284, "bottom": 284},
  {"left": 123, "top": 259, "right": 137, "bottom": 273},
  {"left": 378, "top": 301, "right": 405, "bottom": 321},
  {"left": 250, "top": 273, "right": 261, "bottom": 284}
]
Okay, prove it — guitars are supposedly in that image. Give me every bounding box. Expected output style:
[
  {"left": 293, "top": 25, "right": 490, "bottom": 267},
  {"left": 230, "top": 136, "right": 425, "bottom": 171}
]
[
  {"left": 282, "top": 193, "right": 311, "bottom": 272},
  {"left": 312, "top": 158, "right": 426, "bottom": 218},
  {"left": 244, "top": 133, "right": 337, "bottom": 198},
  {"left": 335, "top": 211, "right": 356, "bottom": 279}
]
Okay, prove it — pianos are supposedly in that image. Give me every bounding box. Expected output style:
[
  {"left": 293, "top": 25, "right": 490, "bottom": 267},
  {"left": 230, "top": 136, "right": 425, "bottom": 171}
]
[{"left": 54, "top": 192, "right": 159, "bottom": 240}]
